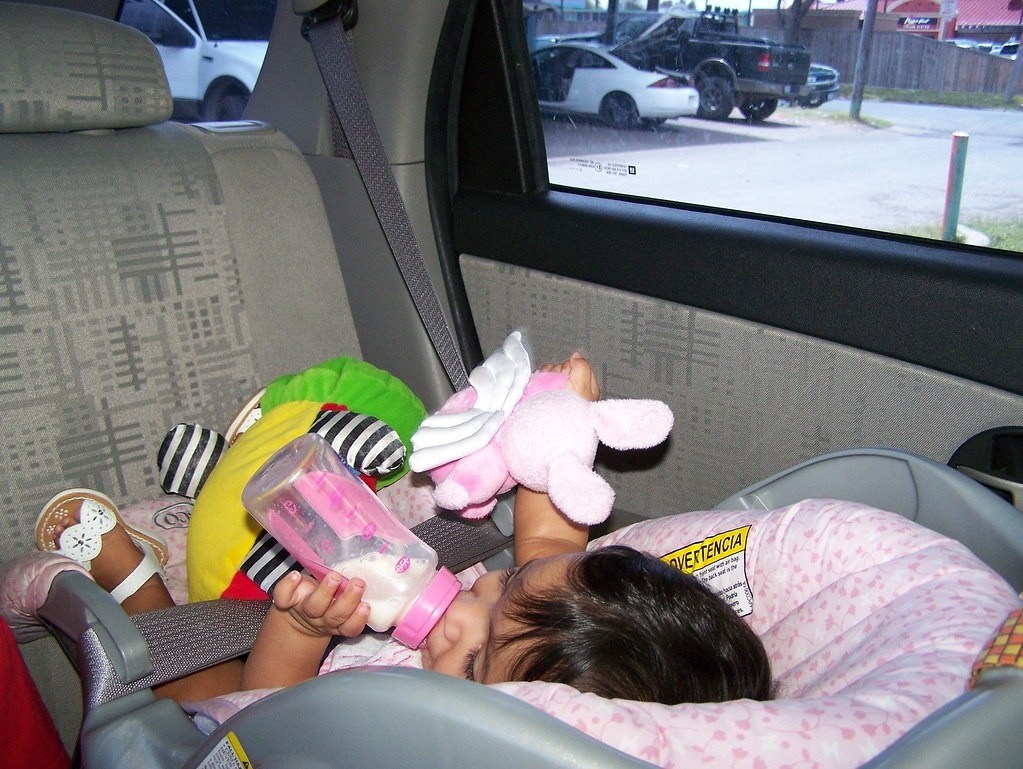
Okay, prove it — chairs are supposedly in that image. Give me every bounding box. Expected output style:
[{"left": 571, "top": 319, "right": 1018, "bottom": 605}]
[{"left": 0, "top": 0, "right": 1023, "bottom": 769}]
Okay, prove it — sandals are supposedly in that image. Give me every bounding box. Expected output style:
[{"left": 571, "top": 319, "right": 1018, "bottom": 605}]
[
  {"left": 223, "top": 385, "right": 268, "bottom": 447},
  {"left": 35, "top": 489, "right": 168, "bottom": 606}
]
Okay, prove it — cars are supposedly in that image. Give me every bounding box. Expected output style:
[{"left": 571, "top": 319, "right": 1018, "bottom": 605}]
[
  {"left": 532, "top": 9, "right": 701, "bottom": 130},
  {"left": 942, "top": 39, "right": 1020, "bottom": 61},
  {"left": 117, "top": 0, "right": 278, "bottom": 121},
  {"left": 797, "top": 64, "right": 839, "bottom": 108}
]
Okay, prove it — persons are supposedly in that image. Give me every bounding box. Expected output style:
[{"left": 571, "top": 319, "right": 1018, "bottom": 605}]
[{"left": 35, "top": 352, "right": 770, "bottom": 705}]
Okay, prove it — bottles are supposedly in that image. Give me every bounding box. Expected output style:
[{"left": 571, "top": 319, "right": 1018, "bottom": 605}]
[{"left": 241, "top": 432, "right": 463, "bottom": 651}]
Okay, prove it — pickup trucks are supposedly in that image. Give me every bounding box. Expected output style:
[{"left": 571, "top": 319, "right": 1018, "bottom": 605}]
[{"left": 614, "top": 4, "right": 811, "bottom": 122}]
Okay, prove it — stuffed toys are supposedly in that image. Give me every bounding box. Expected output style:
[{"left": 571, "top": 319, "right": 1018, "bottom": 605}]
[{"left": 408, "top": 331, "right": 674, "bottom": 526}]
